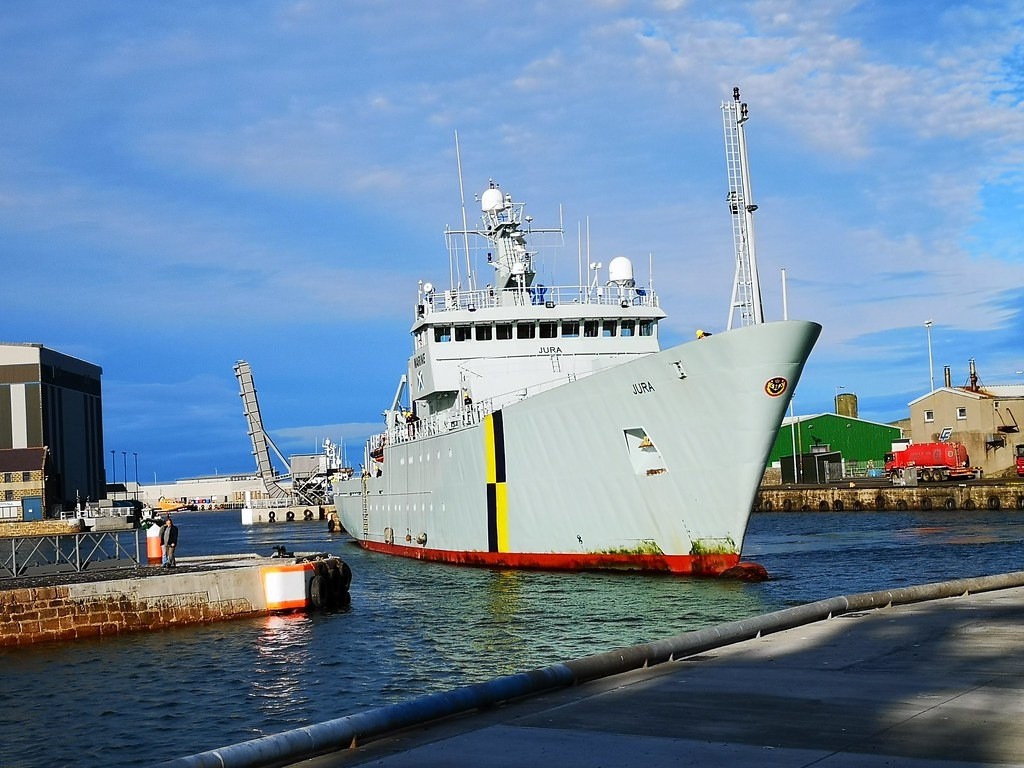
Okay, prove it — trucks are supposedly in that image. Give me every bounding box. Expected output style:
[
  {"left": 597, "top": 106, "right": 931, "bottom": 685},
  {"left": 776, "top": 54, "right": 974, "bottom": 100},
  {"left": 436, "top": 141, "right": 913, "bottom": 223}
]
[{"left": 1013, "top": 444, "right": 1024, "bottom": 477}]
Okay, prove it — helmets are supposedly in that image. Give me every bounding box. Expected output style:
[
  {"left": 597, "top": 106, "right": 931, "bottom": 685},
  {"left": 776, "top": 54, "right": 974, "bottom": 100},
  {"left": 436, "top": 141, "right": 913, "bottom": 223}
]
[{"left": 696, "top": 330, "right": 705, "bottom": 339}]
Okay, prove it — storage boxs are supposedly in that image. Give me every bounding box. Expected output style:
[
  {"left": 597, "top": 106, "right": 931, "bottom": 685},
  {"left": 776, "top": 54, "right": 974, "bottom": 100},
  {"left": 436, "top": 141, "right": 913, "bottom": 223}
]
[{"left": 0, "top": 499, "right": 133, "bottom": 538}]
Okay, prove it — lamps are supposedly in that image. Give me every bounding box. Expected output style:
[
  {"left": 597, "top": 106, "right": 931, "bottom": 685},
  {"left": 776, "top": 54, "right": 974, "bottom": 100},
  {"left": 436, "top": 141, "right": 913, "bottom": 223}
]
[
  {"left": 545, "top": 301, "right": 555, "bottom": 308},
  {"left": 468, "top": 303, "right": 477, "bottom": 312}
]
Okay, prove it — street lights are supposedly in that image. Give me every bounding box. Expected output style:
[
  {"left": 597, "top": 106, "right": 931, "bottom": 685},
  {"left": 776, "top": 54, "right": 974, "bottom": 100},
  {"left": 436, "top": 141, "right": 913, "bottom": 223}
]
[
  {"left": 925, "top": 320, "right": 933, "bottom": 392},
  {"left": 110, "top": 449, "right": 115, "bottom": 500},
  {"left": 132, "top": 452, "right": 139, "bottom": 500},
  {"left": 121, "top": 451, "right": 128, "bottom": 499},
  {"left": 836, "top": 386, "right": 845, "bottom": 414}
]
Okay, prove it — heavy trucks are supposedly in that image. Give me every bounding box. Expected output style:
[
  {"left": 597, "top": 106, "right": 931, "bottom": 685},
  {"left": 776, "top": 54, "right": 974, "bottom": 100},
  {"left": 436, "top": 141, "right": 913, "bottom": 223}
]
[{"left": 882, "top": 441, "right": 983, "bottom": 484}]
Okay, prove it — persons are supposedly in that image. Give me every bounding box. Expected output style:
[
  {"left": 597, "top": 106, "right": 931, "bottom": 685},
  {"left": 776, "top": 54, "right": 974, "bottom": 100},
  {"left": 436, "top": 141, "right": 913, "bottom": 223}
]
[
  {"left": 374, "top": 466, "right": 382, "bottom": 494},
  {"left": 696, "top": 330, "right": 713, "bottom": 340},
  {"left": 403, "top": 412, "right": 422, "bottom": 439},
  {"left": 463, "top": 391, "right": 473, "bottom": 425},
  {"left": 342, "top": 477, "right": 346, "bottom": 481},
  {"left": 361, "top": 469, "right": 371, "bottom": 495},
  {"left": 331, "top": 477, "right": 337, "bottom": 492},
  {"left": 159, "top": 519, "right": 178, "bottom": 568}
]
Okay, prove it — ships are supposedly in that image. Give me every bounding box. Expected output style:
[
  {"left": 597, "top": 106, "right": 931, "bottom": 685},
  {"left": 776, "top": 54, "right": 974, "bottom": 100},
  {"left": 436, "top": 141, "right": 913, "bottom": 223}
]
[{"left": 331, "top": 86, "right": 824, "bottom": 580}]
